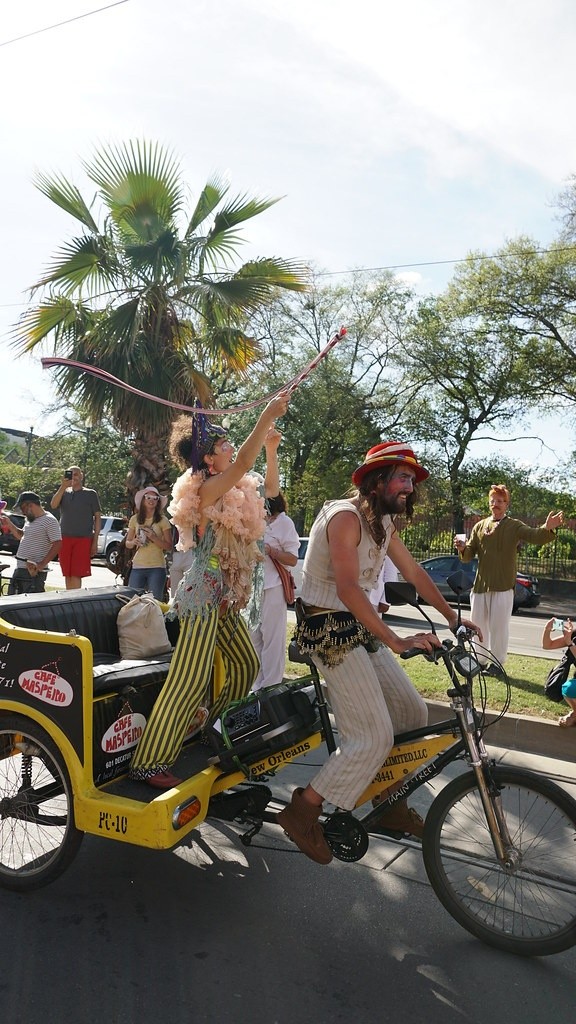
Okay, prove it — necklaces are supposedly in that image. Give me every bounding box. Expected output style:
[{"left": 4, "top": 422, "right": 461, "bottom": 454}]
[
  {"left": 357, "top": 494, "right": 382, "bottom": 549},
  {"left": 484, "top": 514, "right": 506, "bottom": 535}
]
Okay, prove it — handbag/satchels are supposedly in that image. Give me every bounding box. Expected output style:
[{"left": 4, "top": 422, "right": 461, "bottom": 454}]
[
  {"left": 545, "top": 637, "right": 576, "bottom": 704},
  {"left": 274, "top": 562, "right": 296, "bottom": 605},
  {"left": 114, "top": 590, "right": 176, "bottom": 661},
  {"left": 121, "top": 560, "right": 133, "bottom": 586}
]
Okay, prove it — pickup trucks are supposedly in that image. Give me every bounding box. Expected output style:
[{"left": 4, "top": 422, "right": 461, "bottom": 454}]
[{"left": 92, "top": 516, "right": 128, "bottom": 574}]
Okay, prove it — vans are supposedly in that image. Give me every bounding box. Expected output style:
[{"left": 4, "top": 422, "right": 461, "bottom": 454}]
[{"left": 0, "top": 513, "right": 26, "bottom": 555}]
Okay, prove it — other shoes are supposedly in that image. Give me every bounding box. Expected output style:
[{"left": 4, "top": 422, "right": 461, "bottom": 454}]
[
  {"left": 559, "top": 710, "right": 576, "bottom": 728},
  {"left": 145, "top": 769, "right": 182, "bottom": 789},
  {"left": 481, "top": 664, "right": 504, "bottom": 677}
]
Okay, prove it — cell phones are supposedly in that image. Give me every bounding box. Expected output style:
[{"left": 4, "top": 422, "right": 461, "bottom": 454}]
[
  {"left": 553, "top": 619, "right": 564, "bottom": 631},
  {"left": 64, "top": 471, "right": 72, "bottom": 480}
]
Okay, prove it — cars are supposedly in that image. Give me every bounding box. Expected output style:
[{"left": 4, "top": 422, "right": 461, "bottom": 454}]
[
  {"left": 415, "top": 555, "right": 541, "bottom": 615},
  {"left": 286, "top": 537, "right": 310, "bottom": 609}
]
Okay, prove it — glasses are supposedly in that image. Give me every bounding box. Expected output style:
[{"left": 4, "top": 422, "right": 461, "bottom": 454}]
[
  {"left": 144, "top": 493, "right": 161, "bottom": 501},
  {"left": 213, "top": 439, "right": 236, "bottom": 453}
]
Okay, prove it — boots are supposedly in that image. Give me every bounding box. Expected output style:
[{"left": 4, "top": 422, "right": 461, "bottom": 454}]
[
  {"left": 372, "top": 795, "right": 426, "bottom": 838},
  {"left": 276, "top": 786, "right": 334, "bottom": 865}
]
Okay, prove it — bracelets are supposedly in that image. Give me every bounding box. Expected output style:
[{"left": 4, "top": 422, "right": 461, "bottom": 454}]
[{"left": 568, "top": 642, "right": 575, "bottom": 647}]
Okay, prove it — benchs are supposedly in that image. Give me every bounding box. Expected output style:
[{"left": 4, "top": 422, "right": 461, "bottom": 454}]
[{"left": 0, "top": 590, "right": 180, "bottom": 698}]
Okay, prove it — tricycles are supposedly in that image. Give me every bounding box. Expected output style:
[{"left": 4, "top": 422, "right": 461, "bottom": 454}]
[{"left": 0, "top": 571, "right": 576, "bottom": 955}]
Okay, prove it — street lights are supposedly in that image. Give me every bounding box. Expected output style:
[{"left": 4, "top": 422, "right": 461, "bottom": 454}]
[
  {"left": 25, "top": 414, "right": 38, "bottom": 471},
  {"left": 80, "top": 415, "right": 94, "bottom": 488}
]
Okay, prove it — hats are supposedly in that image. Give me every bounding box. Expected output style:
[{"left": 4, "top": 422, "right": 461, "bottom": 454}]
[
  {"left": 0, "top": 500, "right": 7, "bottom": 510},
  {"left": 352, "top": 441, "right": 429, "bottom": 488},
  {"left": 11, "top": 490, "right": 41, "bottom": 509},
  {"left": 135, "top": 486, "right": 168, "bottom": 510},
  {"left": 190, "top": 397, "right": 229, "bottom": 474}
]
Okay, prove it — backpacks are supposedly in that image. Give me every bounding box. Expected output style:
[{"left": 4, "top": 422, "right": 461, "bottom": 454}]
[{"left": 115, "top": 533, "right": 136, "bottom": 574}]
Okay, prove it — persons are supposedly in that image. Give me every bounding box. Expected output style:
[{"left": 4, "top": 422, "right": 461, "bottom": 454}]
[
  {"left": 1, "top": 491, "right": 62, "bottom": 596},
  {"left": 542, "top": 617, "right": 576, "bottom": 728},
  {"left": 453, "top": 485, "right": 563, "bottom": 676},
  {"left": 276, "top": 442, "right": 484, "bottom": 863},
  {"left": 128, "top": 397, "right": 282, "bottom": 790},
  {"left": 125, "top": 486, "right": 173, "bottom": 602},
  {"left": 0, "top": 501, "right": 7, "bottom": 524},
  {"left": 51, "top": 465, "right": 102, "bottom": 590},
  {"left": 246, "top": 490, "right": 301, "bottom": 692}
]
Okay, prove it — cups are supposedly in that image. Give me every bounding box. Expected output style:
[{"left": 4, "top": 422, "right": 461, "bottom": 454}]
[
  {"left": 138, "top": 528, "right": 149, "bottom": 546},
  {"left": 456, "top": 534, "right": 467, "bottom": 546}
]
